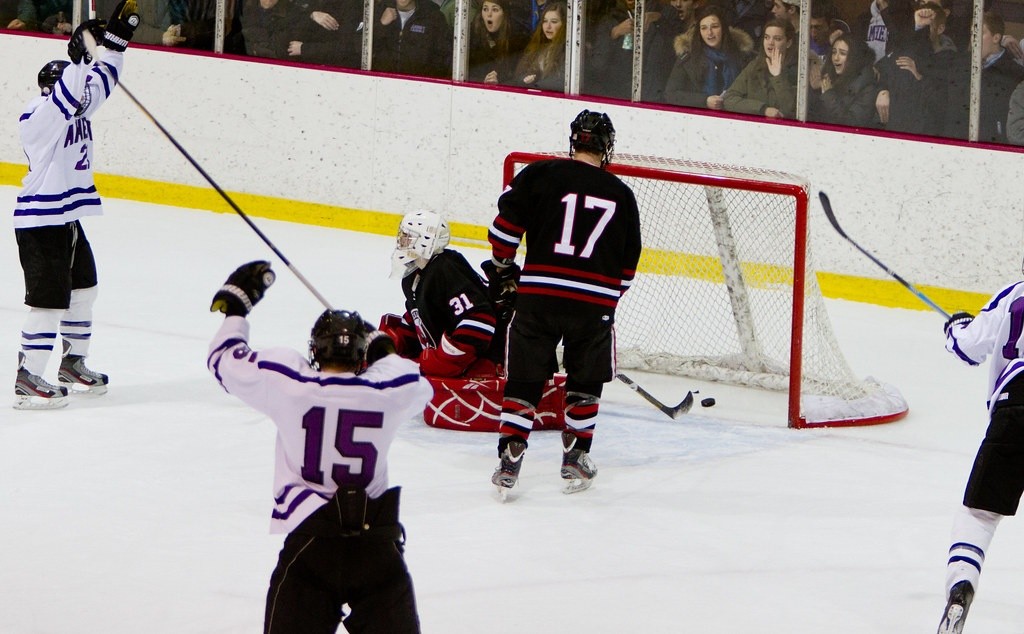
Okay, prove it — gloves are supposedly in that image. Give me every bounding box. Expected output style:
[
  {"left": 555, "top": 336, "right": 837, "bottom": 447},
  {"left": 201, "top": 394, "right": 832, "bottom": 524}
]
[
  {"left": 103, "top": 0, "right": 140, "bottom": 53},
  {"left": 211, "top": 261, "right": 275, "bottom": 316},
  {"left": 481, "top": 261, "right": 520, "bottom": 308},
  {"left": 364, "top": 322, "right": 393, "bottom": 364},
  {"left": 162, "top": 24, "right": 186, "bottom": 47},
  {"left": 944, "top": 313, "right": 975, "bottom": 335},
  {"left": 67, "top": 18, "right": 106, "bottom": 65}
]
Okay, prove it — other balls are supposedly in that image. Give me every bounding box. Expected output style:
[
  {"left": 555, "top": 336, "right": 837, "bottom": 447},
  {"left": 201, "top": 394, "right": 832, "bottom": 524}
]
[{"left": 701, "top": 397, "right": 716, "bottom": 407}]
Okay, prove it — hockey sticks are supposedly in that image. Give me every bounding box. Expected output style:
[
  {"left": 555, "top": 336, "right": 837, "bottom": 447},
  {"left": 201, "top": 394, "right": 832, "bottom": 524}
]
[
  {"left": 616, "top": 370, "right": 696, "bottom": 421},
  {"left": 817, "top": 187, "right": 955, "bottom": 321},
  {"left": 82, "top": 29, "right": 334, "bottom": 313}
]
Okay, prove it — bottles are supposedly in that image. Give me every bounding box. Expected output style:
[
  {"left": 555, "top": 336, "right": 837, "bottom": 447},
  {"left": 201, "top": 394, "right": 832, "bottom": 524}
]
[{"left": 621, "top": 33, "right": 634, "bottom": 51}]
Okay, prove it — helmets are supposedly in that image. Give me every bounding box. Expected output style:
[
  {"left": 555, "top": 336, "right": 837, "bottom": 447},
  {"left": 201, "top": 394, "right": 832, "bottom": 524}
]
[
  {"left": 570, "top": 109, "right": 615, "bottom": 169},
  {"left": 310, "top": 310, "right": 366, "bottom": 376},
  {"left": 391, "top": 211, "right": 450, "bottom": 264},
  {"left": 38, "top": 60, "right": 70, "bottom": 94}
]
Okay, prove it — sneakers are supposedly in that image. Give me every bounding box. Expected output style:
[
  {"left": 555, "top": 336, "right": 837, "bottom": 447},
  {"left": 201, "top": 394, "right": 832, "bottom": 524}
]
[
  {"left": 561, "top": 433, "right": 597, "bottom": 494},
  {"left": 14, "top": 351, "right": 69, "bottom": 409},
  {"left": 937, "top": 581, "right": 974, "bottom": 634},
  {"left": 492, "top": 435, "right": 528, "bottom": 500},
  {"left": 58, "top": 339, "right": 108, "bottom": 395}
]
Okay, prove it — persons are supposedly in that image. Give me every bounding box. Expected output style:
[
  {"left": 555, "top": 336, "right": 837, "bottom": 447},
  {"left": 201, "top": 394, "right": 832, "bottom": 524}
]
[
  {"left": 936, "top": 259, "right": 1024, "bottom": 634},
  {"left": 486, "top": 109, "right": 642, "bottom": 488},
  {"left": 466, "top": 0, "right": 1024, "bottom": 146},
  {"left": 13, "top": 0, "right": 140, "bottom": 397},
  {"left": 206, "top": 258, "right": 434, "bottom": 633},
  {"left": 0, "top": 0, "right": 452, "bottom": 80},
  {"left": 380, "top": 210, "right": 561, "bottom": 377}
]
